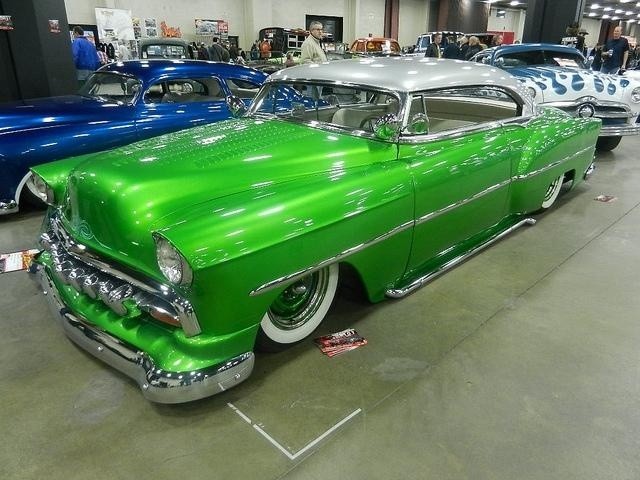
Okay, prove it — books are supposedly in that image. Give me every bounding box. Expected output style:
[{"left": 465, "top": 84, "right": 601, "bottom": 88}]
[
  {"left": 313, "top": 328, "right": 369, "bottom": 357},
  {"left": 0, "top": 248, "right": 43, "bottom": 273}
]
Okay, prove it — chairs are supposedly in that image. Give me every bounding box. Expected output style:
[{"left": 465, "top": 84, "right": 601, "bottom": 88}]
[{"left": 334, "top": 90, "right": 477, "bottom": 140}]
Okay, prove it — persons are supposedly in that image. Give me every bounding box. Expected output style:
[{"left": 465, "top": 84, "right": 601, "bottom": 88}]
[
  {"left": 197, "top": 37, "right": 245, "bottom": 63},
  {"left": 589, "top": 26, "right": 629, "bottom": 75},
  {"left": 71, "top": 26, "right": 101, "bottom": 87},
  {"left": 559, "top": 23, "right": 588, "bottom": 56},
  {"left": 425, "top": 32, "right": 503, "bottom": 61},
  {"left": 300, "top": 21, "right": 329, "bottom": 65},
  {"left": 285, "top": 52, "right": 294, "bottom": 66},
  {"left": 249, "top": 38, "right": 272, "bottom": 60}
]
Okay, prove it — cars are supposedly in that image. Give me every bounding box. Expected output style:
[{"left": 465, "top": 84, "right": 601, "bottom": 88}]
[
  {"left": 0, "top": 60, "right": 331, "bottom": 216},
  {"left": 258, "top": 27, "right": 310, "bottom": 60},
  {"left": 26, "top": 57, "right": 604, "bottom": 404},
  {"left": 452, "top": 42, "right": 640, "bottom": 152},
  {"left": 402, "top": 31, "right": 465, "bottom": 58},
  {"left": 343, "top": 37, "right": 403, "bottom": 59},
  {"left": 138, "top": 37, "right": 191, "bottom": 59}
]
